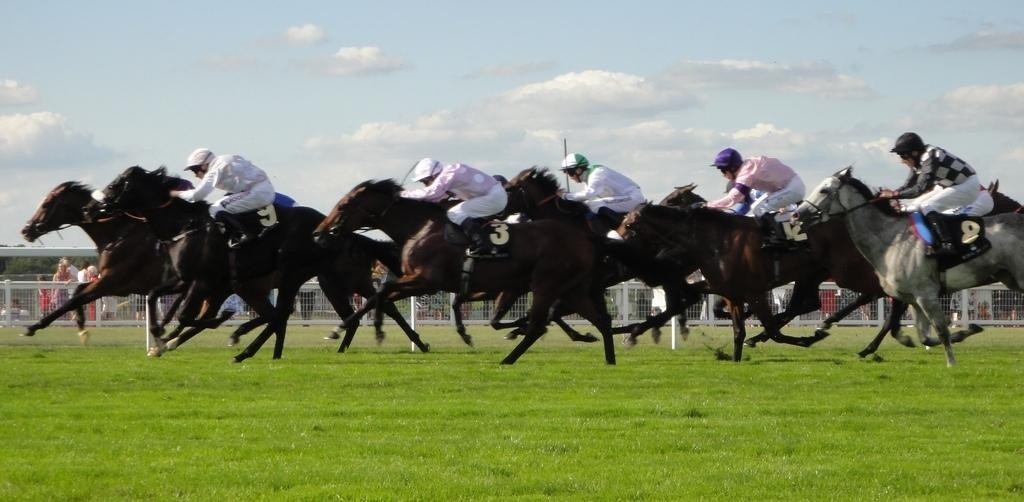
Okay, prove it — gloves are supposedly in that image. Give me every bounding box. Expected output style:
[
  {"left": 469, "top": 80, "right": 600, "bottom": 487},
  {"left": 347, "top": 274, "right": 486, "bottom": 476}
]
[{"left": 691, "top": 202, "right": 705, "bottom": 210}]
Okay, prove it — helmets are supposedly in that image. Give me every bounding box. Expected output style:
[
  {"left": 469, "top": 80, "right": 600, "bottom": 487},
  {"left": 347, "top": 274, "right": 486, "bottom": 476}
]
[
  {"left": 184, "top": 148, "right": 215, "bottom": 171},
  {"left": 711, "top": 148, "right": 742, "bottom": 167},
  {"left": 890, "top": 133, "right": 926, "bottom": 153},
  {"left": 413, "top": 158, "right": 442, "bottom": 182},
  {"left": 560, "top": 153, "right": 589, "bottom": 170}
]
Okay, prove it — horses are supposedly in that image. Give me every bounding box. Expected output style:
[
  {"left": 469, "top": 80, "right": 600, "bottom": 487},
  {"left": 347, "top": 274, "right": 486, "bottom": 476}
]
[
  {"left": 790, "top": 165, "right": 1024, "bottom": 369},
  {"left": 978, "top": 178, "right": 1023, "bottom": 216},
  {"left": 20, "top": 181, "right": 209, "bottom": 361},
  {"left": 311, "top": 179, "right": 646, "bottom": 365},
  {"left": 492, "top": 166, "right": 641, "bottom": 344},
  {"left": 657, "top": 182, "right": 822, "bottom": 348},
  {"left": 81, "top": 163, "right": 430, "bottom": 365},
  {"left": 819, "top": 220, "right": 916, "bottom": 359},
  {"left": 616, "top": 199, "right": 831, "bottom": 363}
]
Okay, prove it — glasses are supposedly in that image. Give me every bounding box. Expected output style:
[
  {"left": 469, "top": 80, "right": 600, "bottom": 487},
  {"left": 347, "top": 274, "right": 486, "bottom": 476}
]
[
  {"left": 190, "top": 166, "right": 201, "bottom": 172},
  {"left": 421, "top": 179, "right": 432, "bottom": 184},
  {"left": 567, "top": 170, "right": 577, "bottom": 177},
  {"left": 721, "top": 169, "right": 729, "bottom": 174},
  {"left": 900, "top": 154, "right": 912, "bottom": 160}
]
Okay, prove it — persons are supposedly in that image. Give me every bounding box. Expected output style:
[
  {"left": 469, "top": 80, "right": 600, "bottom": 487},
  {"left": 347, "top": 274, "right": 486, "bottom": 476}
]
[
  {"left": 877, "top": 132, "right": 995, "bottom": 260},
  {"left": 40, "top": 255, "right": 1024, "bottom": 326},
  {"left": 555, "top": 153, "right": 649, "bottom": 246},
  {"left": 391, "top": 157, "right": 510, "bottom": 260},
  {"left": 691, "top": 147, "right": 806, "bottom": 252},
  {"left": 168, "top": 148, "right": 276, "bottom": 249}
]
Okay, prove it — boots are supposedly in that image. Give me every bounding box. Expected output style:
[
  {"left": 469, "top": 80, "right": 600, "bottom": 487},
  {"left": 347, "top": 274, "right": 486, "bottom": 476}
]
[
  {"left": 460, "top": 216, "right": 491, "bottom": 257},
  {"left": 597, "top": 207, "right": 623, "bottom": 225},
  {"left": 214, "top": 210, "right": 249, "bottom": 248},
  {"left": 760, "top": 211, "right": 779, "bottom": 249},
  {"left": 925, "top": 211, "right": 955, "bottom": 257}
]
